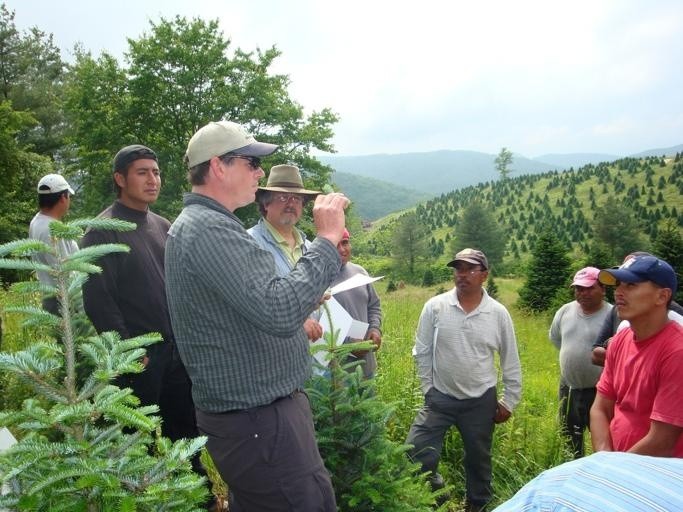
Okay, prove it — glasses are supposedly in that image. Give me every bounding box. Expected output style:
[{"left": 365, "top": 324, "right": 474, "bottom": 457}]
[{"left": 227, "top": 155, "right": 260, "bottom": 167}]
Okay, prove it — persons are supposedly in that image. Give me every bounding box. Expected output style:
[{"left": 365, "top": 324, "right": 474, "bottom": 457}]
[
  {"left": 27, "top": 174, "right": 78, "bottom": 344},
  {"left": 589, "top": 299, "right": 682, "bottom": 367},
  {"left": 79, "top": 145, "right": 223, "bottom": 512},
  {"left": 246, "top": 165, "right": 332, "bottom": 394},
  {"left": 163, "top": 121, "right": 351, "bottom": 512},
  {"left": 549, "top": 267, "right": 614, "bottom": 458},
  {"left": 616, "top": 251, "right": 682, "bottom": 331},
  {"left": 403, "top": 248, "right": 522, "bottom": 511},
  {"left": 326, "top": 231, "right": 381, "bottom": 398},
  {"left": 590, "top": 250, "right": 682, "bottom": 457}
]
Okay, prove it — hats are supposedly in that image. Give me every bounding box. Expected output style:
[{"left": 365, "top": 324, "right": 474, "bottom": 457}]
[
  {"left": 37, "top": 174, "right": 75, "bottom": 196},
  {"left": 113, "top": 145, "right": 156, "bottom": 171},
  {"left": 570, "top": 251, "right": 677, "bottom": 291},
  {"left": 185, "top": 121, "right": 278, "bottom": 170},
  {"left": 447, "top": 248, "right": 488, "bottom": 270},
  {"left": 258, "top": 165, "right": 323, "bottom": 195},
  {"left": 343, "top": 229, "right": 350, "bottom": 241}
]
